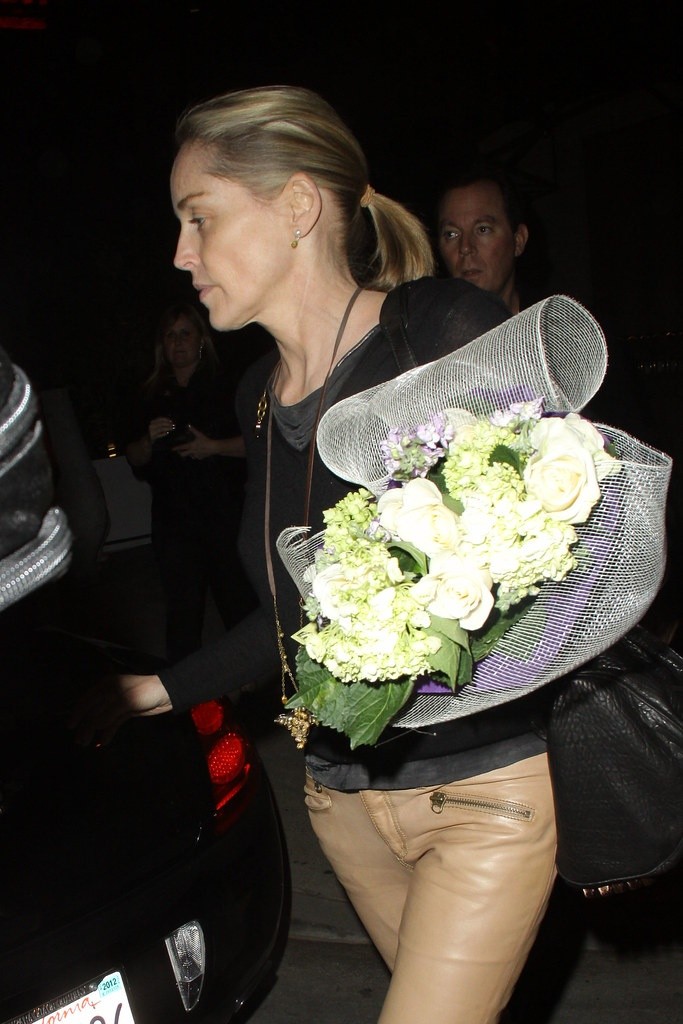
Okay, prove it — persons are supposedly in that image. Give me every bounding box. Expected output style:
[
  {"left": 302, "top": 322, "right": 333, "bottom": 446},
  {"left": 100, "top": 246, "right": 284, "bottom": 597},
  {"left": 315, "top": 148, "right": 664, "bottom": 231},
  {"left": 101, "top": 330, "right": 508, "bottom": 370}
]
[
  {"left": 124, "top": 303, "right": 262, "bottom": 666},
  {"left": 437, "top": 172, "right": 529, "bottom": 316},
  {"left": 65, "top": 86, "right": 595, "bottom": 1024},
  {"left": 0, "top": 271, "right": 110, "bottom": 578}
]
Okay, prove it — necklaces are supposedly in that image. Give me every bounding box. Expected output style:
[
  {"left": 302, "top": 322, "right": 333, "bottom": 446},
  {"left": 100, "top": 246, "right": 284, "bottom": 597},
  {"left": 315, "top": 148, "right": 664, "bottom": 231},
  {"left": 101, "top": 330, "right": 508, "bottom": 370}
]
[{"left": 256, "top": 288, "right": 367, "bottom": 750}]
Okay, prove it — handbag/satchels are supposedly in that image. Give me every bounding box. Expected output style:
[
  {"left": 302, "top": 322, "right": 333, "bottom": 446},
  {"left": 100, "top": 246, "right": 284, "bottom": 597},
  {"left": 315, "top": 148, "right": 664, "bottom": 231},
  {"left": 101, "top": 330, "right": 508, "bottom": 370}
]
[{"left": 543, "top": 621, "right": 683, "bottom": 907}]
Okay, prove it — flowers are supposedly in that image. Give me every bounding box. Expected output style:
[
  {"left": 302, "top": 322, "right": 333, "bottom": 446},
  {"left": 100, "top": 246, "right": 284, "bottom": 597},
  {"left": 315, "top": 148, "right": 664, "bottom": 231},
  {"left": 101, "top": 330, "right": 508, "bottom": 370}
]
[{"left": 274, "top": 407, "right": 613, "bottom": 752}]
